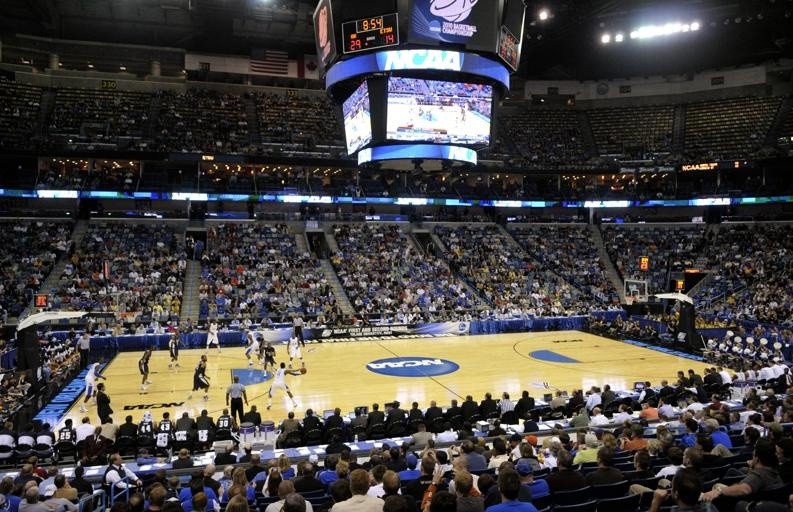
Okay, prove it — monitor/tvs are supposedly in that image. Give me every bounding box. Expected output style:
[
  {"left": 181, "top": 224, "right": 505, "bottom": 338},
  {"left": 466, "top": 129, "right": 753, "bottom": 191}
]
[
  {"left": 349, "top": 412, "right": 356, "bottom": 419},
  {"left": 305, "top": 412, "right": 316, "bottom": 418},
  {"left": 323, "top": 410, "right": 334, "bottom": 420},
  {"left": 356, "top": 407, "right": 368, "bottom": 414},
  {"left": 384, "top": 403, "right": 394, "bottom": 417},
  {"left": 633, "top": 382, "right": 644, "bottom": 392}
]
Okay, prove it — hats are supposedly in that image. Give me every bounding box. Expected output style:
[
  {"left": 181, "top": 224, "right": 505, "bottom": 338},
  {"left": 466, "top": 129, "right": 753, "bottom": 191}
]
[
  {"left": 406, "top": 450, "right": 418, "bottom": 469},
  {"left": 514, "top": 459, "right": 532, "bottom": 474},
  {"left": 772, "top": 437, "right": 793, "bottom": 451},
  {"left": 761, "top": 418, "right": 783, "bottom": 434},
  {"left": 524, "top": 436, "right": 538, "bottom": 446}
]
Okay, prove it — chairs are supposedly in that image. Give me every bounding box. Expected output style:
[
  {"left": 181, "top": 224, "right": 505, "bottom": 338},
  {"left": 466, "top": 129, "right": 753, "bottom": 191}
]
[
  {"left": 0, "top": 340, "right": 80, "bottom": 427},
  {"left": 701, "top": 314, "right": 792, "bottom": 387},
  {"left": 589, "top": 313, "right": 657, "bottom": 343}
]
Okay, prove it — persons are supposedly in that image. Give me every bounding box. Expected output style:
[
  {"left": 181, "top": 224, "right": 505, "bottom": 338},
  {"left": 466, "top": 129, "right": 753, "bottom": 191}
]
[{"left": 1, "top": 85, "right": 793, "bottom": 510}]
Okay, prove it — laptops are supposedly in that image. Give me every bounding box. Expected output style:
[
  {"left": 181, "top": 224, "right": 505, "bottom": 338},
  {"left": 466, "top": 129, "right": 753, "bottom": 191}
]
[{"left": 543, "top": 394, "right": 551, "bottom": 403}]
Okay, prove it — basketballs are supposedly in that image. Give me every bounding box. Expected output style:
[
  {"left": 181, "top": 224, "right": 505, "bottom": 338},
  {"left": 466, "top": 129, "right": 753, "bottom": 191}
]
[{"left": 299, "top": 368, "right": 305, "bottom": 372}]
